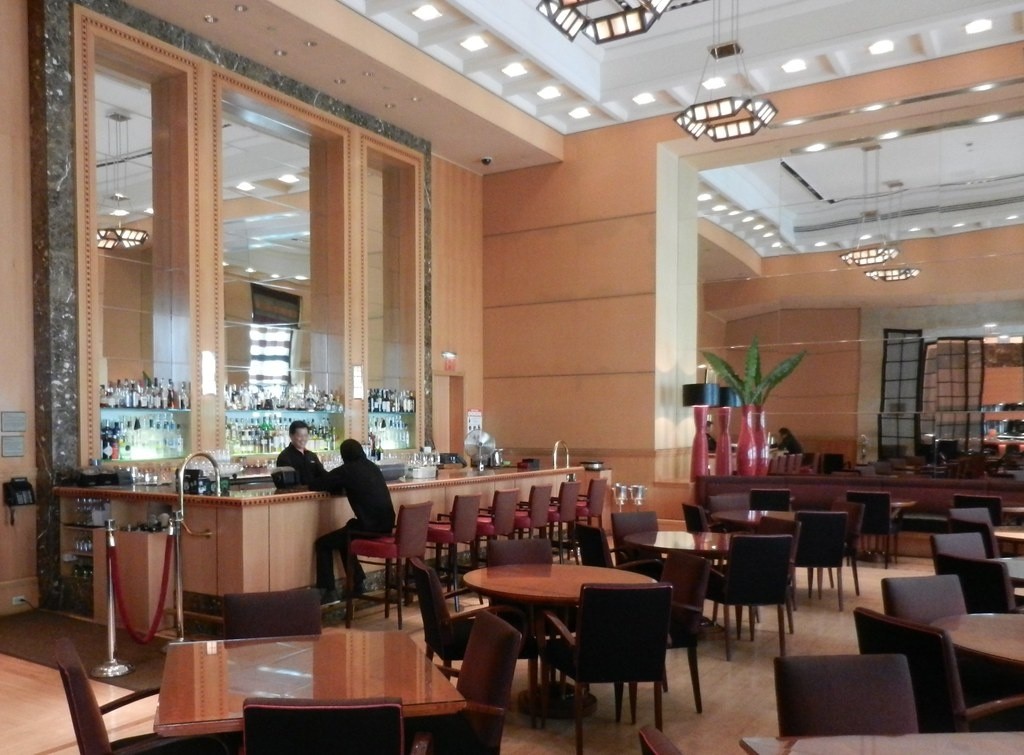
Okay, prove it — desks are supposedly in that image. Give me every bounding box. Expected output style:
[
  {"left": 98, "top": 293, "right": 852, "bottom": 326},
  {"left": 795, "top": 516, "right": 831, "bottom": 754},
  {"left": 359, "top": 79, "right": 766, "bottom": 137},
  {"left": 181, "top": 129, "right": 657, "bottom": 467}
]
[
  {"left": 710, "top": 510, "right": 794, "bottom": 531},
  {"left": 708, "top": 446, "right": 789, "bottom": 476},
  {"left": 929, "top": 612, "right": 1024, "bottom": 693},
  {"left": 463, "top": 563, "right": 658, "bottom": 720},
  {"left": 989, "top": 556, "right": 1024, "bottom": 589},
  {"left": 993, "top": 525, "right": 1024, "bottom": 557},
  {"left": 622, "top": 529, "right": 746, "bottom": 641},
  {"left": 738, "top": 731, "right": 1024, "bottom": 755},
  {"left": 856, "top": 497, "right": 918, "bottom": 563},
  {"left": 1002, "top": 506, "right": 1024, "bottom": 526},
  {"left": 153, "top": 630, "right": 468, "bottom": 738}
]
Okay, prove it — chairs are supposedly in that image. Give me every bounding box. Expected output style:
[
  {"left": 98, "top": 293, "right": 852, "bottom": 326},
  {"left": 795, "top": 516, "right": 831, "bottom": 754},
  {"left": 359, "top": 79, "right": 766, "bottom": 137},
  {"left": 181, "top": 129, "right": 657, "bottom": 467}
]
[
  {"left": 54, "top": 479, "right": 1024, "bottom": 755},
  {"left": 767, "top": 439, "right": 1024, "bottom": 479}
]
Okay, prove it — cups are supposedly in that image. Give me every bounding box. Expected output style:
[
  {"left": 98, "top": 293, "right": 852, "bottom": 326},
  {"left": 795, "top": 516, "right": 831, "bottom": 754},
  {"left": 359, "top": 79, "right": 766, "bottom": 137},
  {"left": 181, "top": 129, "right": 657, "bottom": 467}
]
[
  {"left": 182, "top": 449, "right": 240, "bottom": 477},
  {"left": 383, "top": 451, "right": 440, "bottom": 466}
]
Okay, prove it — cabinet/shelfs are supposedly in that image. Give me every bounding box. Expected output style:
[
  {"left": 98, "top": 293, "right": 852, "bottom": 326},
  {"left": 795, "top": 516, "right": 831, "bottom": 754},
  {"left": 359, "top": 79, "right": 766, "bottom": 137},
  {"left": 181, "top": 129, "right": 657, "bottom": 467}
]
[
  {"left": 369, "top": 412, "right": 416, "bottom": 450},
  {"left": 100, "top": 407, "right": 192, "bottom": 464},
  {"left": 224, "top": 409, "right": 344, "bottom": 458}
]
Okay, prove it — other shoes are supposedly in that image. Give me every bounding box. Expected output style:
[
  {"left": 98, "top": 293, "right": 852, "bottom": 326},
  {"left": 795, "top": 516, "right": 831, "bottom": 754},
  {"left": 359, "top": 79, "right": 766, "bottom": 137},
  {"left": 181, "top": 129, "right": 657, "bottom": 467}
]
[
  {"left": 320, "top": 589, "right": 341, "bottom": 606},
  {"left": 350, "top": 579, "right": 370, "bottom": 598}
]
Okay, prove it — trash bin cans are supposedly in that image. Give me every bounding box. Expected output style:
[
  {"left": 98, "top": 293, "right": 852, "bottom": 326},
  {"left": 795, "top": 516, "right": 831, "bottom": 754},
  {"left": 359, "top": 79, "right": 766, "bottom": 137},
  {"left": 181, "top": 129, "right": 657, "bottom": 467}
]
[{"left": 115, "top": 529, "right": 174, "bottom": 633}]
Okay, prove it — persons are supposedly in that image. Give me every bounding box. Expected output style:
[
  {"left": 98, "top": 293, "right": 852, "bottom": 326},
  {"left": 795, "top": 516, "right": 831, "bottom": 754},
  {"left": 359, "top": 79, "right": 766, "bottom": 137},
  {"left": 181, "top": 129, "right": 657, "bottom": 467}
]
[
  {"left": 274, "top": 421, "right": 329, "bottom": 486},
  {"left": 773, "top": 427, "right": 803, "bottom": 455},
  {"left": 310, "top": 439, "right": 397, "bottom": 606},
  {"left": 706, "top": 421, "right": 717, "bottom": 451}
]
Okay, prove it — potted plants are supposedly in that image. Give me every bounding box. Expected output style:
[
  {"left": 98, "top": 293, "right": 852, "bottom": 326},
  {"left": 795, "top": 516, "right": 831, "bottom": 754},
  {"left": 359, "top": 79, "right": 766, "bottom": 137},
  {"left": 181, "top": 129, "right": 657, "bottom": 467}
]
[
  {"left": 712, "top": 344, "right": 803, "bottom": 477},
  {"left": 700, "top": 336, "right": 805, "bottom": 476}
]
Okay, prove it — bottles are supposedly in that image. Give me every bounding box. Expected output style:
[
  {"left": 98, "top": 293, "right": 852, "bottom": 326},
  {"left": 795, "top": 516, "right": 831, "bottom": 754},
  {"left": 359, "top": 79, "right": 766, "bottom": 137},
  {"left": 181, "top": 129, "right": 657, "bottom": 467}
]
[
  {"left": 225, "top": 382, "right": 344, "bottom": 453},
  {"left": 98, "top": 377, "right": 190, "bottom": 461},
  {"left": 368, "top": 386, "right": 415, "bottom": 448}
]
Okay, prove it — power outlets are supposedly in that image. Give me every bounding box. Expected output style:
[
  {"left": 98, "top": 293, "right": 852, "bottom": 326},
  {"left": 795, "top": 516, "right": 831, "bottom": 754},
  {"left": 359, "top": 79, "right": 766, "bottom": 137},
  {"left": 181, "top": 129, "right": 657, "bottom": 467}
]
[{"left": 12, "top": 596, "right": 26, "bottom": 606}]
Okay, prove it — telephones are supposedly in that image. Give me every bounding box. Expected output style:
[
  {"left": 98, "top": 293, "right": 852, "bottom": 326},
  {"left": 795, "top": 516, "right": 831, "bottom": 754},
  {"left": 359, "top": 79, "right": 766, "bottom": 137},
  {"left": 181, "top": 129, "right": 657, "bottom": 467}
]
[{"left": 2, "top": 477, "right": 36, "bottom": 507}]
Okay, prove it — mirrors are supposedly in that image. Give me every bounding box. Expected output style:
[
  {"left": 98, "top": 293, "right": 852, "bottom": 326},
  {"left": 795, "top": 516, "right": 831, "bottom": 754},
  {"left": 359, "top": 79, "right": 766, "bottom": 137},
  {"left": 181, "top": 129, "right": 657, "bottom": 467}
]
[{"left": 73, "top": 2, "right": 426, "bottom": 475}]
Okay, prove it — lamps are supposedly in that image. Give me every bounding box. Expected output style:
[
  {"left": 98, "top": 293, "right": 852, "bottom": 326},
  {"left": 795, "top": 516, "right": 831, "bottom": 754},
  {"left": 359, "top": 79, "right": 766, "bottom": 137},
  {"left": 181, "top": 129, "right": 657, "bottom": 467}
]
[
  {"left": 837, "top": 143, "right": 901, "bottom": 268},
  {"left": 863, "top": 177, "right": 921, "bottom": 283},
  {"left": 708, "top": 387, "right": 743, "bottom": 476},
  {"left": 673, "top": 0, "right": 779, "bottom": 143},
  {"left": 682, "top": 383, "right": 720, "bottom": 482},
  {"left": 536, "top": 0, "right": 674, "bottom": 46},
  {"left": 97, "top": 107, "right": 150, "bottom": 251}
]
